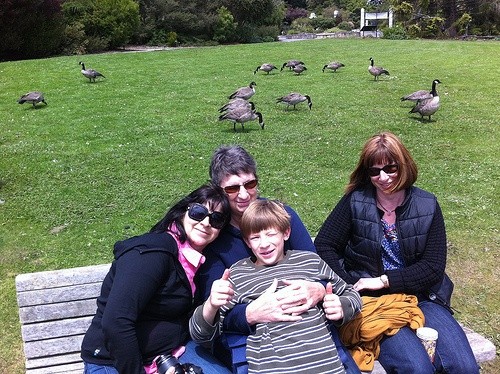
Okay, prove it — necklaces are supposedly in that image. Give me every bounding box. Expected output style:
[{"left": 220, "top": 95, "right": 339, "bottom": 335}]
[{"left": 376, "top": 198, "right": 396, "bottom": 216}]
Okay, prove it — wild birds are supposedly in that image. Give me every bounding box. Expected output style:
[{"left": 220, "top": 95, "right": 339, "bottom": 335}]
[
  {"left": 280, "top": 61, "right": 307, "bottom": 75},
  {"left": 275, "top": 93, "right": 312, "bottom": 111},
  {"left": 78, "top": 61, "right": 105, "bottom": 83},
  {"left": 322, "top": 62, "right": 345, "bottom": 73},
  {"left": 400, "top": 79, "right": 442, "bottom": 122},
  {"left": 228, "top": 82, "right": 257, "bottom": 101},
  {"left": 367, "top": 57, "right": 390, "bottom": 80},
  {"left": 217, "top": 98, "right": 265, "bottom": 132},
  {"left": 254, "top": 64, "right": 278, "bottom": 75},
  {"left": 18, "top": 92, "right": 47, "bottom": 109}
]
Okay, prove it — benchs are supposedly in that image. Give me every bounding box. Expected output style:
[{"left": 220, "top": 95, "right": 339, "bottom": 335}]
[{"left": 14, "top": 238, "right": 496, "bottom": 374}]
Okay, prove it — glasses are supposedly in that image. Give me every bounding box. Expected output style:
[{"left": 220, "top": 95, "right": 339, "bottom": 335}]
[
  {"left": 366, "top": 164, "right": 399, "bottom": 177},
  {"left": 221, "top": 173, "right": 258, "bottom": 193},
  {"left": 187, "top": 202, "right": 227, "bottom": 229}
]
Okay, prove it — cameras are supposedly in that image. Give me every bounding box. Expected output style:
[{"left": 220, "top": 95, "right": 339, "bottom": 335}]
[{"left": 155, "top": 353, "right": 185, "bottom": 374}]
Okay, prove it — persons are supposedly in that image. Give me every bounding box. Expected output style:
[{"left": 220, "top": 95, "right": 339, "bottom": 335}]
[
  {"left": 313, "top": 134, "right": 481, "bottom": 374},
  {"left": 193, "top": 145, "right": 363, "bottom": 374},
  {"left": 81, "top": 185, "right": 233, "bottom": 374},
  {"left": 188, "top": 199, "right": 363, "bottom": 374}
]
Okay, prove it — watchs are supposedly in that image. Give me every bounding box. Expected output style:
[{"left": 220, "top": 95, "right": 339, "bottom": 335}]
[{"left": 380, "top": 275, "right": 389, "bottom": 288}]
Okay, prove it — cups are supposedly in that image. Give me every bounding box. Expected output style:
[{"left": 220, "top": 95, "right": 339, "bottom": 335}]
[{"left": 416, "top": 327, "right": 439, "bottom": 363}]
